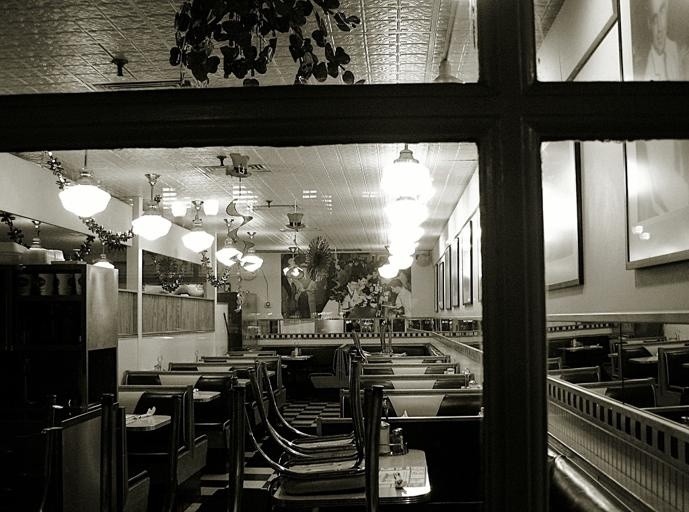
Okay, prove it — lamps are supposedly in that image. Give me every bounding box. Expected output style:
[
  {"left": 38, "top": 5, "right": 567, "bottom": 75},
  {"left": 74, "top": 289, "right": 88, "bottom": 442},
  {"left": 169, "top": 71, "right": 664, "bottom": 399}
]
[
  {"left": 184, "top": 199, "right": 215, "bottom": 254},
  {"left": 60, "top": 150, "right": 111, "bottom": 217},
  {"left": 215, "top": 219, "right": 242, "bottom": 265},
  {"left": 93, "top": 243, "right": 114, "bottom": 269},
  {"left": 432, "top": 61, "right": 461, "bottom": 82},
  {"left": 240, "top": 232, "right": 263, "bottom": 271},
  {"left": 284, "top": 248, "right": 302, "bottom": 276},
  {"left": 132, "top": 174, "right": 172, "bottom": 241},
  {"left": 393, "top": 143, "right": 418, "bottom": 163}
]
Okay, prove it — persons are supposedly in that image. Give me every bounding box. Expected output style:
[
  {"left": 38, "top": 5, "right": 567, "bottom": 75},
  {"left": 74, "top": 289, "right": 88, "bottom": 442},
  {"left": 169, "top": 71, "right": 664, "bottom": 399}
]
[
  {"left": 636, "top": 0, "right": 689, "bottom": 216},
  {"left": 389, "top": 280, "right": 411, "bottom": 318},
  {"left": 357, "top": 276, "right": 368, "bottom": 295},
  {"left": 343, "top": 277, "right": 367, "bottom": 308}
]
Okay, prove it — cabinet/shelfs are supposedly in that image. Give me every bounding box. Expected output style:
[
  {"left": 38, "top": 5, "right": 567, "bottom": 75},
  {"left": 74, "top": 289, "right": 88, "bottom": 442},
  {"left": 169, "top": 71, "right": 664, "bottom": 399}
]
[{"left": 0, "top": 264, "right": 118, "bottom": 512}]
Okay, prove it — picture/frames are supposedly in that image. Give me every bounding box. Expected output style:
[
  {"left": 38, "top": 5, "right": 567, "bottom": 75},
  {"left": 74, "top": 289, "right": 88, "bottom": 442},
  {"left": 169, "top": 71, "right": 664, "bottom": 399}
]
[
  {"left": 462, "top": 220, "right": 472, "bottom": 304},
  {"left": 451, "top": 237, "right": 459, "bottom": 306},
  {"left": 434, "top": 263, "right": 438, "bottom": 311},
  {"left": 617, "top": 1, "right": 687, "bottom": 271},
  {"left": 542, "top": 143, "right": 582, "bottom": 290},
  {"left": 445, "top": 245, "right": 451, "bottom": 310},
  {"left": 439, "top": 262, "right": 443, "bottom": 309}
]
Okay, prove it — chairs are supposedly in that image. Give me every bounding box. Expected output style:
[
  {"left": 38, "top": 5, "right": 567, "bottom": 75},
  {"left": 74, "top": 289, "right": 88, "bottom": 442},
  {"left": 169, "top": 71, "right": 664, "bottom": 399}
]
[
  {"left": 250, "top": 361, "right": 362, "bottom": 464},
  {"left": 228, "top": 385, "right": 384, "bottom": 512},
  {"left": 231, "top": 370, "right": 368, "bottom": 494}
]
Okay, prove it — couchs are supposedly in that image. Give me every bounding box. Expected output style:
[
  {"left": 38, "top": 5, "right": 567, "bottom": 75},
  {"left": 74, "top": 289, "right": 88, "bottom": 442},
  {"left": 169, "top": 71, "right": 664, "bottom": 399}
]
[
  {"left": 547, "top": 357, "right": 562, "bottom": 370},
  {"left": 340, "top": 389, "right": 483, "bottom": 416},
  {"left": 340, "top": 344, "right": 349, "bottom": 388},
  {"left": 310, "top": 345, "right": 340, "bottom": 388},
  {"left": 282, "top": 385, "right": 286, "bottom": 403},
  {"left": 122, "top": 371, "right": 232, "bottom": 475},
  {"left": 170, "top": 363, "right": 261, "bottom": 440},
  {"left": 350, "top": 373, "right": 473, "bottom": 389},
  {"left": 641, "top": 405, "right": 688, "bottom": 462},
  {"left": 579, "top": 378, "right": 657, "bottom": 409},
  {"left": 118, "top": 384, "right": 209, "bottom": 504},
  {"left": 118, "top": 385, "right": 191, "bottom": 512},
  {"left": 657, "top": 347, "right": 689, "bottom": 406},
  {"left": 202, "top": 356, "right": 280, "bottom": 414},
  {"left": 318, "top": 417, "right": 480, "bottom": 502},
  {"left": 261, "top": 396, "right": 270, "bottom": 433},
  {"left": 547, "top": 366, "right": 600, "bottom": 383},
  {"left": 618, "top": 339, "right": 688, "bottom": 379},
  {"left": 362, "top": 362, "right": 459, "bottom": 374},
  {"left": 366, "top": 357, "right": 450, "bottom": 362},
  {"left": 118, "top": 407, "right": 149, "bottom": 512}
]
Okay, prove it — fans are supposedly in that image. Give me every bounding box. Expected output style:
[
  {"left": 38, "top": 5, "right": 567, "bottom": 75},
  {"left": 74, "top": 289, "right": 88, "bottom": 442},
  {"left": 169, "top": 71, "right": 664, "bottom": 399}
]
[
  {"left": 279, "top": 197, "right": 318, "bottom": 232},
  {"left": 213, "top": 153, "right": 271, "bottom": 197}
]
